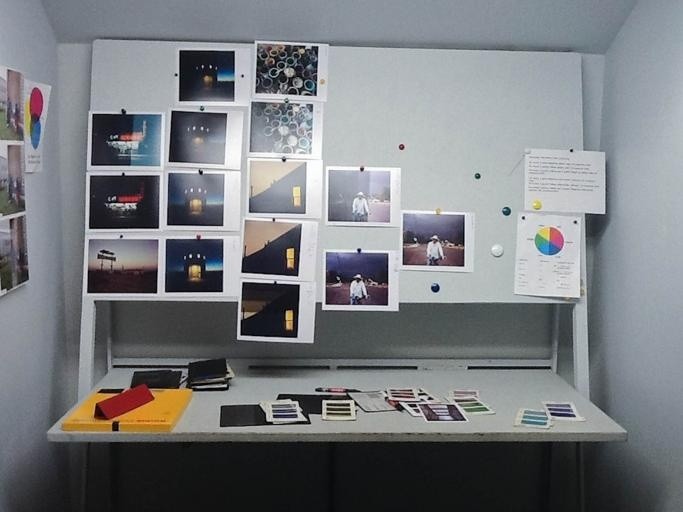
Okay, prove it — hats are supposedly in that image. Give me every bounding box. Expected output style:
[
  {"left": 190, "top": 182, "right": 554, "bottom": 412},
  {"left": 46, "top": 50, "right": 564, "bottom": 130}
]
[
  {"left": 356, "top": 192, "right": 366, "bottom": 198},
  {"left": 430, "top": 235, "right": 439, "bottom": 241},
  {"left": 352, "top": 274, "right": 363, "bottom": 280}
]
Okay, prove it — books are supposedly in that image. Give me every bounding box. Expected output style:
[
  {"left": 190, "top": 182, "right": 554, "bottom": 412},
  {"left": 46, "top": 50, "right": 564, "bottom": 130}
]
[
  {"left": 187, "top": 358, "right": 228, "bottom": 384},
  {"left": 186, "top": 382, "right": 228, "bottom": 391}
]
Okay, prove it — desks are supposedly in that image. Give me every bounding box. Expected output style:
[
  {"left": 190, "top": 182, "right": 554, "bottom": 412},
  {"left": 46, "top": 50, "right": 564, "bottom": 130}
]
[{"left": 48, "top": 366, "right": 628, "bottom": 512}]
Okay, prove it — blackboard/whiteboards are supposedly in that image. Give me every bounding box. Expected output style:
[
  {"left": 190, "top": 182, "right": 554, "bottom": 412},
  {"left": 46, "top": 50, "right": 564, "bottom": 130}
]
[{"left": 87, "top": 42, "right": 585, "bottom": 303}]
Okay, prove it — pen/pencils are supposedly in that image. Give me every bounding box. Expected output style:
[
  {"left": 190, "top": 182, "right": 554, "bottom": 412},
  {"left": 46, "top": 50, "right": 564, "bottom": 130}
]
[
  {"left": 386, "top": 400, "right": 403, "bottom": 413},
  {"left": 315, "top": 387, "right": 362, "bottom": 392}
]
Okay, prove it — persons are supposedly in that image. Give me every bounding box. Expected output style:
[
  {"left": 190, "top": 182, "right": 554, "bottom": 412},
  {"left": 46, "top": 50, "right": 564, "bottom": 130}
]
[
  {"left": 423, "top": 232, "right": 447, "bottom": 265},
  {"left": 348, "top": 273, "right": 371, "bottom": 305},
  {"left": 349, "top": 190, "right": 369, "bottom": 223}
]
